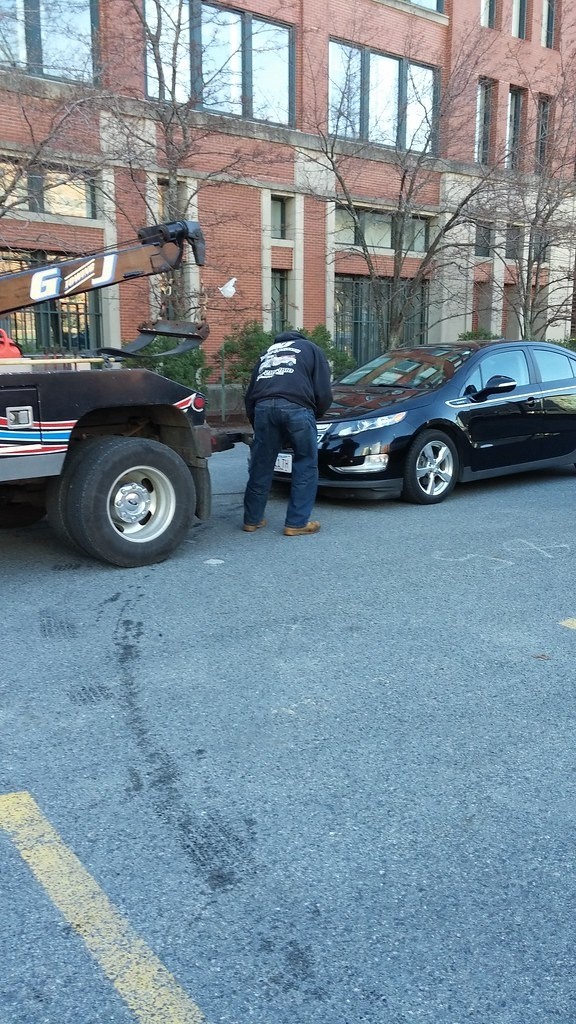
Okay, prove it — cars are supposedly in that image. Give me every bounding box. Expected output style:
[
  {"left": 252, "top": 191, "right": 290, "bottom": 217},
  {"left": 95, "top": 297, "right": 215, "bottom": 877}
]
[{"left": 248, "top": 340, "right": 576, "bottom": 504}]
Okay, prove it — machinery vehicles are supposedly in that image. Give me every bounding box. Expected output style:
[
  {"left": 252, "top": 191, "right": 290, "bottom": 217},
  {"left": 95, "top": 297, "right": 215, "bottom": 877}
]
[{"left": 0, "top": 220, "right": 212, "bottom": 567}]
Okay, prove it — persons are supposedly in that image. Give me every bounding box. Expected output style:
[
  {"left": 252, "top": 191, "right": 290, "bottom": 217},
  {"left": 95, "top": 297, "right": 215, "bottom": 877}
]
[{"left": 243, "top": 332, "right": 333, "bottom": 537}]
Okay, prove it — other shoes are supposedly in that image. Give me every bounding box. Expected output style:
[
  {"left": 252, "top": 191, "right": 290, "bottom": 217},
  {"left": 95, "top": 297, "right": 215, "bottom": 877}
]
[
  {"left": 284, "top": 521, "right": 321, "bottom": 535},
  {"left": 243, "top": 519, "right": 267, "bottom": 531}
]
